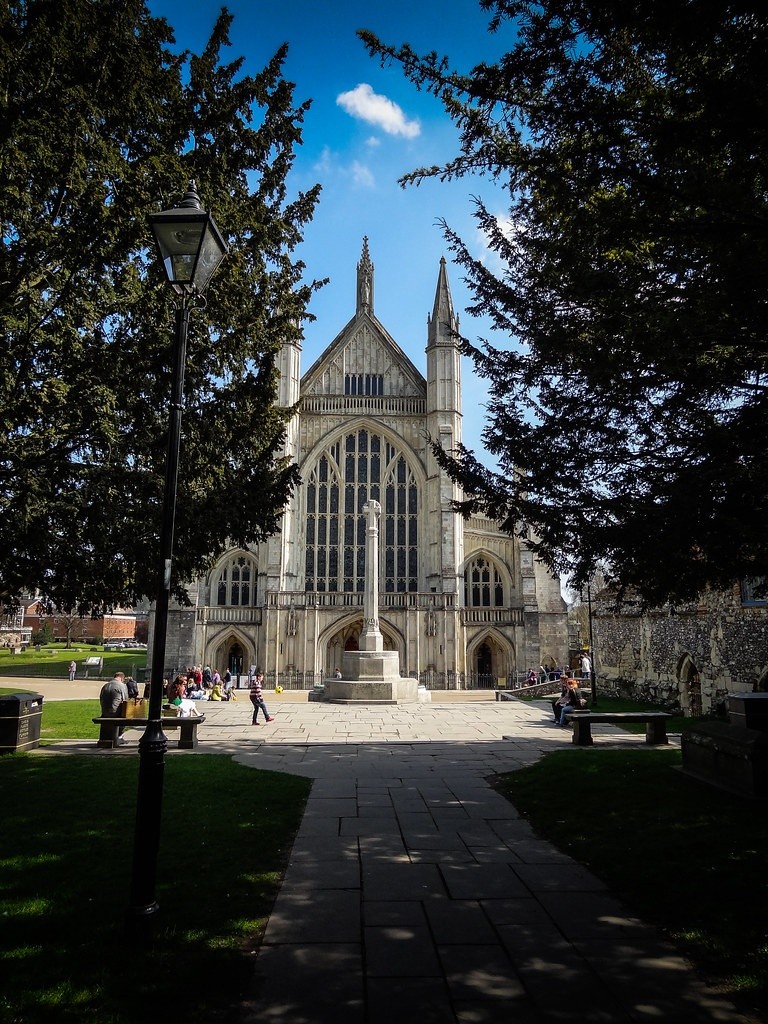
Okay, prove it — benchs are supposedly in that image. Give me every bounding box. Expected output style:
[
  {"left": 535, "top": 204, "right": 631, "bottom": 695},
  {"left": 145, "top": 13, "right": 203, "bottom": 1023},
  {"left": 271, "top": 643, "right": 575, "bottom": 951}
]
[
  {"left": 162, "top": 708, "right": 180, "bottom": 730},
  {"left": 563, "top": 713, "right": 673, "bottom": 746},
  {"left": 93, "top": 717, "right": 205, "bottom": 749}
]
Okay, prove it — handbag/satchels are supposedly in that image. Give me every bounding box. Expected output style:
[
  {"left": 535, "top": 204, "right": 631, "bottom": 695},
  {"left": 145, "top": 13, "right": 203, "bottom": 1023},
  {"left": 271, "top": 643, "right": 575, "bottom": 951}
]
[
  {"left": 579, "top": 699, "right": 587, "bottom": 707},
  {"left": 126, "top": 692, "right": 149, "bottom": 718}
]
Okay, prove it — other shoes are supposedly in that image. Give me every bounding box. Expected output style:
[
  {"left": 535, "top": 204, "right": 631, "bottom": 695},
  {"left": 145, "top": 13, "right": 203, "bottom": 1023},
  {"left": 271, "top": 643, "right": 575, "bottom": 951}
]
[
  {"left": 551, "top": 718, "right": 565, "bottom": 727},
  {"left": 198, "top": 713, "right": 204, "bottom": 717},
  {"left": 266, "top": 717, "right": 274, "bottom": 722},
  {"left": 252, "top": 722, "right": 259, "bottom": 725}
]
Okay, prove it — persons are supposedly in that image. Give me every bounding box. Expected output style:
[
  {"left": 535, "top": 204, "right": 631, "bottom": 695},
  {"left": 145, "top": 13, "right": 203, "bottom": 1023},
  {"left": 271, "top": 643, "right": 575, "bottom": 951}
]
[
  {"left": 524, "top": 653, "right": 592, "bottom": 728},
  {"left": 66, "top": 659, "right": 77, "bottom": 682},
  {"left": 248, "top": 672, "right": 275, "bottom": 725},
  {"left": 99, "top": 671, "right": 128, "bottom": 740},
  {"left": 126, "top": 662, "right": 241, "bottom": 718},
  {"left": 334, "top": 668, "right": 342, "bottom": 679}
]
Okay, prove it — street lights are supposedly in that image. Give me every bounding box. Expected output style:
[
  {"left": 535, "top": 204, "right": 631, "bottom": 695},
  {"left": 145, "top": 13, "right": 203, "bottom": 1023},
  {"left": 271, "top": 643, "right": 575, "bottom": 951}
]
[{"left": 132, "top": 176, "right": 236, "bottom": 908}]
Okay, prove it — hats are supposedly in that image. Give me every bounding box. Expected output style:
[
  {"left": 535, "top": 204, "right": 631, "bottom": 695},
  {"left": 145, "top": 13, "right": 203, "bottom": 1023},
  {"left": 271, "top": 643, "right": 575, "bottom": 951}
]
[
  {"left": 567, "top": 680, "right": 576, "bottom": 685},
  {"left": 560, "top": 674, "right": 568, "bottom": 678}
]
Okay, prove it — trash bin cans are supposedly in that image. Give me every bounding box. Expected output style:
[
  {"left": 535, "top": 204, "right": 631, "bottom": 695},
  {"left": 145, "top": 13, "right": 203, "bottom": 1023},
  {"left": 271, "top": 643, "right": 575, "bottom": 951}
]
[{"left": 0, "top": 692, "right": 45, "bottom": 753}]
